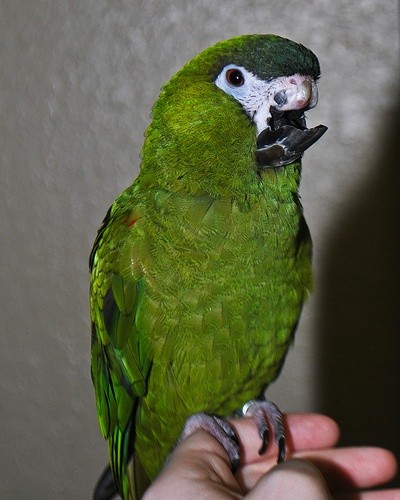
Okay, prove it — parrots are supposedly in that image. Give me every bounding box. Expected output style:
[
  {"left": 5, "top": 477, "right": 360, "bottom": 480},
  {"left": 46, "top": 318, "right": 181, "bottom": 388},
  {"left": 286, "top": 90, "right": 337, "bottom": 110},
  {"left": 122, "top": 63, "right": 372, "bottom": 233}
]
[{"left": 89, "top": 34, "right": 327, "bottom": 500}]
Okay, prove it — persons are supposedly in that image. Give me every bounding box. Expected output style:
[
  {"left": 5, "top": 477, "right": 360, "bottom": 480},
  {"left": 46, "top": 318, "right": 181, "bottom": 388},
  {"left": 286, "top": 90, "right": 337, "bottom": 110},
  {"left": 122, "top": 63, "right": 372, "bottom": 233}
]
[{"left": 136, "top": 408, "right": 399, "bottom": 500}]
